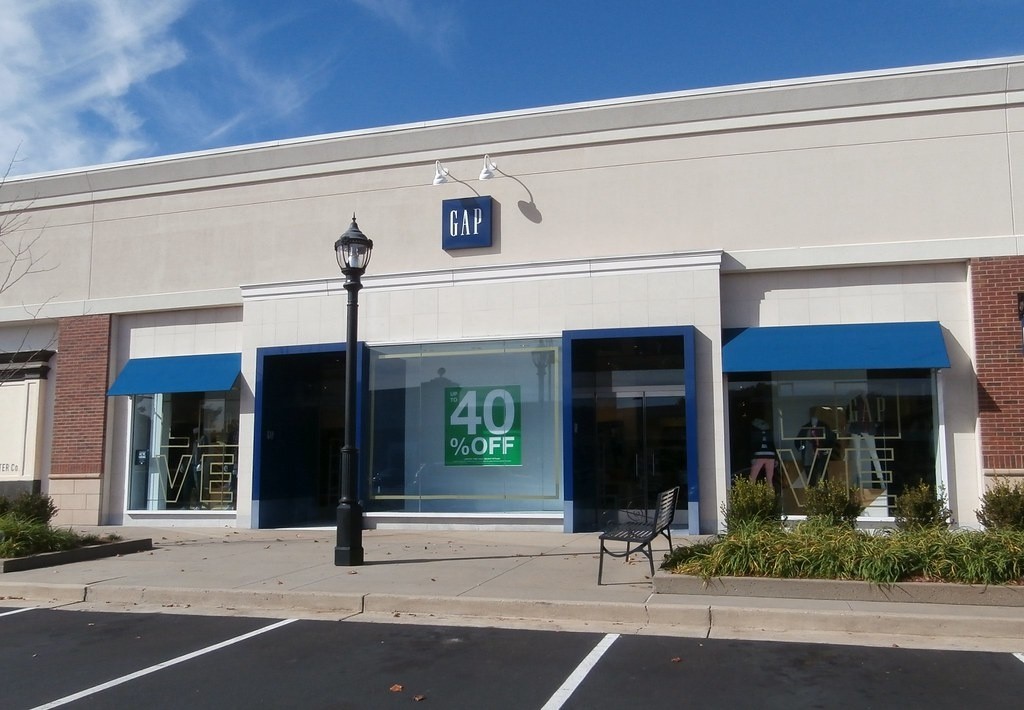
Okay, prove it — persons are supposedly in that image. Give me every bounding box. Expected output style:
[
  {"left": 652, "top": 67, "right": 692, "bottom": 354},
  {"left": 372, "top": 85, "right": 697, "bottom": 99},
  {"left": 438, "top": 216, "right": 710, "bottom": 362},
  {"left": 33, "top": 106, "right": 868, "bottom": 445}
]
[
  {"left": 749, "top": 418, "right": 779, "bottom": 490},
  {"left": 794, "top": 406, "right": 831, "bottom": 489},
  {"left": 845, "top": 390, "right": 886, "bottom": 491}
]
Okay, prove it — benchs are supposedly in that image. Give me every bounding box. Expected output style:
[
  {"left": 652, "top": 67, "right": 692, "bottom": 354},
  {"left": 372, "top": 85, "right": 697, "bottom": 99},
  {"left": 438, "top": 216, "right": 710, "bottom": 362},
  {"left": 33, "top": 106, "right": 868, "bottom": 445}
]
[{"left": 598, "top": 486, "right": 679, "bottom": 585}]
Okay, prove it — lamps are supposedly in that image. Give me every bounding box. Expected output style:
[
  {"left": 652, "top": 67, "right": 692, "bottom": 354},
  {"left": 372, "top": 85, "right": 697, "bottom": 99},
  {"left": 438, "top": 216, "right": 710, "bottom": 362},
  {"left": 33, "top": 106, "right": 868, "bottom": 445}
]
[
  {"left": 433, "top": 154, "right": 448, "bottom": 185},
  {"left": 479, "top": 155, "right": 496, "bottom": 180}
]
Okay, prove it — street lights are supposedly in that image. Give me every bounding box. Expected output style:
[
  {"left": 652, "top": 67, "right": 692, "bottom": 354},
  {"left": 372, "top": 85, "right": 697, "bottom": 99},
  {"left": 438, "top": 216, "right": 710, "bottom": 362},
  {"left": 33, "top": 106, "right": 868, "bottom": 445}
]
[{"left": 334, "top": 210, "right": 373, "bottom": 567}]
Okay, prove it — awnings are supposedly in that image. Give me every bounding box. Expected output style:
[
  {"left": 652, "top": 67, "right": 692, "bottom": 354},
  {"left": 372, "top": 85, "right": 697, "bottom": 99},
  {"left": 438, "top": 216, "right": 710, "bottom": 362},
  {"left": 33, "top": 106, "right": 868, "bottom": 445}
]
[
  {"left": 723, "top": 321, "right": 950, "bottom": 370},
  {"left": 106, "top": 353, "right": 242, "bottom": 396}
]
